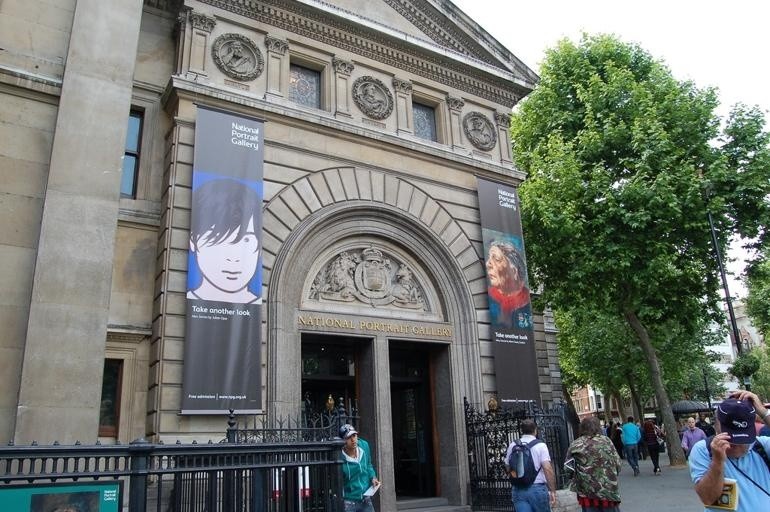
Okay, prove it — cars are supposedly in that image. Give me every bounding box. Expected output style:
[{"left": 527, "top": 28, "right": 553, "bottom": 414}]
[{"left": 754, "top": 402, "right": 770, "bottom": 437}]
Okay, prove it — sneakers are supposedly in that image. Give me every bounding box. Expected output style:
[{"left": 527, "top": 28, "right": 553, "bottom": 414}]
[
  {"left": 633, "top": 465, "right": 639, "bottom": 477},
  {"left": 654, "top": 467, "right": 660, "bottom": 476}
]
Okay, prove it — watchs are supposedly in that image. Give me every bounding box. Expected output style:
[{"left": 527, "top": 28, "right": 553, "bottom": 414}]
[{"left": 762, "top": 408, "right": 770, "bottom": 420}]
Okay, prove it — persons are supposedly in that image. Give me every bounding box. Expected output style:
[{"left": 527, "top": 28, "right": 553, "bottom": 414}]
[
  {"left": 486, "top": 239, "right": 532, "bottom": 331},
  {"left": 337, "top": 424, "right": 379, "bottom": 512},
  {"left": 471, "top": 120, "right": 488, "bottom": 143},
  {"left": 187, "top": 180, "right": 260, "bottom": 305},
  {"left": 360, "top": 85, "right": 383, "bottom": 110},
  {"left": 606, "top": 414, "right": 717, "bottom": 475},
  {"left": 503, "top": 419, "right": 557, "bottom": 512},
  {"left": 564, "top": 416, "right": 621, "bottom": 512},
  {"left": 223, "top": 42, "right": 250, "bottom": 72},
  {"left": 687, "top": 389, "right": 770, "bottom": 512}
]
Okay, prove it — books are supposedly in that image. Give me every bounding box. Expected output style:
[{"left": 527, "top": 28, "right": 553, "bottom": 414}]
[{"left": 704, "top": 479, "right": 738, "bottom": 511}]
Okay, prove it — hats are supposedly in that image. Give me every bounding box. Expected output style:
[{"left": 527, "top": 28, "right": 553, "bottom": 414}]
[
  {"left": 716, "top": 397, "right": 758, "bottom": 446},
  {"left": 338, "top": 423, "right": 359, "bottom": 439}
]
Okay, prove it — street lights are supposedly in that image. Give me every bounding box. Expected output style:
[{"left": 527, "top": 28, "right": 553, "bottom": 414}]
[
  {"left": 587, "top": 378, "right": 600, "bottom": 427},
  {"left": 702, "top": 366, "right": 712, "bottom": 407},
  {"left": 693, "top": 168, "right": 757, "bottom": 407}
]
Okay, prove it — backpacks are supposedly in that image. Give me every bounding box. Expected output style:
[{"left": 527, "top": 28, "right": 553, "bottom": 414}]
[{"left": 507, "top": 438, "right": 546, "bottom": 490}]
